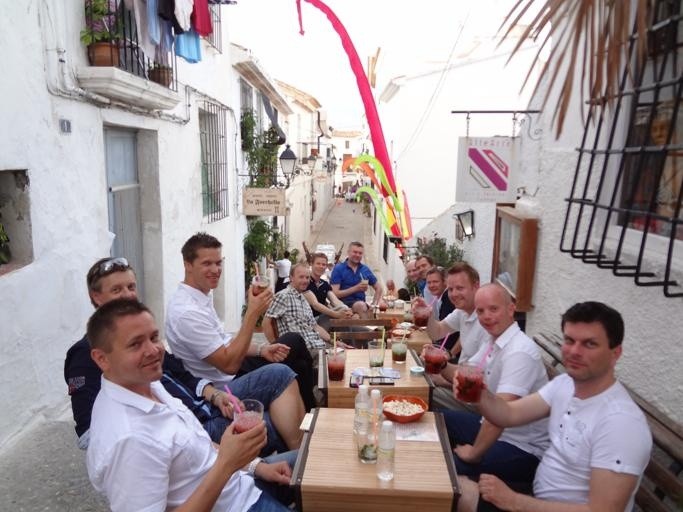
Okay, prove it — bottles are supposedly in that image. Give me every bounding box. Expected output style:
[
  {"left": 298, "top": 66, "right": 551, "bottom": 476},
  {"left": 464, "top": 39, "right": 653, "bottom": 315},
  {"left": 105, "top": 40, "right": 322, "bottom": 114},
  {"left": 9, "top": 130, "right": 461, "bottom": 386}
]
[
  {"left": 354, "top": 385, "right": 367, "bottom": 435},
  {"left": 376, "top": 422, "right": 395, "bottom": 479},
  {"left": 367, "top": 388, "right": 380, "bottom": 439}
]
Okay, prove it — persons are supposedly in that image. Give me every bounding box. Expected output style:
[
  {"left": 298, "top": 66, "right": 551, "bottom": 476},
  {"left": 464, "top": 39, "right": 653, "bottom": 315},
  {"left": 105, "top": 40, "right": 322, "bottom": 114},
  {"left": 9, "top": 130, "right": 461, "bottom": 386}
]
[{"left": 633, "top": 104, "right": 683, "bottom": 240}]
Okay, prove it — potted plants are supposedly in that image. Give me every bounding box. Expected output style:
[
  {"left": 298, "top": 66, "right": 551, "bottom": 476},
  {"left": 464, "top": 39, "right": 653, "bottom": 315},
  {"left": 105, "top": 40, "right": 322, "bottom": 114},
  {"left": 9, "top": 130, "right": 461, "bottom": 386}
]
[{"left": 77, "top": 0, "right": 125, "bottom": 66}]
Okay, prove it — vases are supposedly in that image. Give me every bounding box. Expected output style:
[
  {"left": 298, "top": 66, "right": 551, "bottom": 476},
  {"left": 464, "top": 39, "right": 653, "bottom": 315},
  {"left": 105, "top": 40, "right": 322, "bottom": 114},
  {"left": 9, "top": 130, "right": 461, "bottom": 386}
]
[{"left": 145, "top": 65, "right": 175, "bottom": 88}]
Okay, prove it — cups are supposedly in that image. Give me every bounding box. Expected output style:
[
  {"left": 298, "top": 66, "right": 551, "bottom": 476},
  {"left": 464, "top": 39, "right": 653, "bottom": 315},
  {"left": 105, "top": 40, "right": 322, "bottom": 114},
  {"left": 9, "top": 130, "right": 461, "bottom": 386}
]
[
  {"left": 251, "top": 275, "right": 269, "bottom": 296},
  {"left": 367, "top": 341, "right": 385, "bottom": 367},
  {"left": 413, "top": 306, "right": 428, "bottom": 329},
  {"left": 375, "top": 296, "right": 405, "bottom": 312},
  {"left": 233, "top": 399, "right": 263, "bottom": 433},
  {"left": 328, "top": 349, "right": 346, "bottom": 383},
  {"left": 391, "top": 336, "right": 407, "bottom": 364},
  {"left": 409, "top": 292, "right": 423, "bottom": 313},
  {"left": 424, "top": 343, "right": 445, "bottom": 376},
  {"left": 353, "top": 413, "right": 382, "bottom": 464},
  {"left": 458, "top": 360, "right": 483, "bottom": 403},
  {"left": 359, "top": 280, "right": 368, "bottom": 291}
]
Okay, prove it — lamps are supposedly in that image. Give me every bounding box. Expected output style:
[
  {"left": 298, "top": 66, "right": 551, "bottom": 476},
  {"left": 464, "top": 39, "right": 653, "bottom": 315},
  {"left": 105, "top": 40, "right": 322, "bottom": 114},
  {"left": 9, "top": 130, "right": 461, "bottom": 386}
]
[
  {"left": 264, "top": 145, "right": 298, "bottom": 190},
  {"left": 453, "top": 205, "right": 476, "bottom": 242},
  {"left": 321, "top": 155, "right": 337, "bottom": 170},
  {"left": 296, "top": 153, "right": 315, "bottom": 176}
]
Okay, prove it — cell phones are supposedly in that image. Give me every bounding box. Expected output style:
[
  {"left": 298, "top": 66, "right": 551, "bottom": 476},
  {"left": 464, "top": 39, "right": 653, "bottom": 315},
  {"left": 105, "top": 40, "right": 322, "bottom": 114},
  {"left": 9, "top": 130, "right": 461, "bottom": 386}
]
[
  {"left": 350, "top": 375, "right": 364, "bottom": 388},
  {"left": 369, "top": 377, "right": 394, "bottom": 385}
]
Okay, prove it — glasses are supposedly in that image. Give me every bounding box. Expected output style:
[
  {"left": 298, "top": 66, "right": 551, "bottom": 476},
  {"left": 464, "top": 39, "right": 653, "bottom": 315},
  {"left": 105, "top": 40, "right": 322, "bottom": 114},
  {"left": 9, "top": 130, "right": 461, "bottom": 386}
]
[{"left": 88, "top": 257, "right": 129, "bottom": 285}]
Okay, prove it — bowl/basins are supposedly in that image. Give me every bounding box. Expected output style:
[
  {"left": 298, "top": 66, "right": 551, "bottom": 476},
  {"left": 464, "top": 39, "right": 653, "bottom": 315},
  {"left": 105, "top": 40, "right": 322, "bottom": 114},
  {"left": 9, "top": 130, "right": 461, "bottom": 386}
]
[
  {"left": 382, "top": 394, "right": 428, "bottom": 423},
  {"left": 391, "top": 322, "right": 415, "bottom": 337}
]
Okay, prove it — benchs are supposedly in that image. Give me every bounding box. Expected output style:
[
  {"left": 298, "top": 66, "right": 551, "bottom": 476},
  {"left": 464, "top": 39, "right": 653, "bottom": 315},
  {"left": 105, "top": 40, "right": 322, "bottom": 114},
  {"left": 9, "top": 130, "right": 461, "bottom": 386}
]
[{"left": 530, "top": 325, "right": 683, "bottom": 511}]
